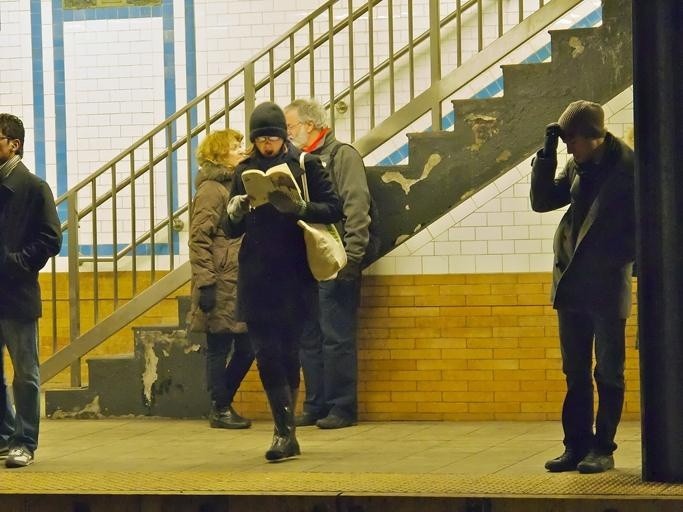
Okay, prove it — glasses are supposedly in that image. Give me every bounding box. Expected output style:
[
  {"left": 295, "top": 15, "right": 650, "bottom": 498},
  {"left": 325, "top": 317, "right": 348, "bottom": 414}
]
[{"left": 254, "top": 136, "right": 280, "bottom": 143}]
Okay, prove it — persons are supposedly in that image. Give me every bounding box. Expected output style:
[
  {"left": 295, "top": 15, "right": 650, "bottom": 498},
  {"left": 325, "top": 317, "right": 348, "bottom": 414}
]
[
  {"left": 528, "top": 100, "right": 635, "bottom": 473},
  {"left": 187, "top": 126, "right": 260, "bottom": 430},
  {"left": 0, "top": 111, "right": 64, "bottom": 469},
  {"left": 281, "top": 96, "right": 373, "bottom": 431},
  {"left": 219, "top": 100, "right": 345, "bottom": 461}
]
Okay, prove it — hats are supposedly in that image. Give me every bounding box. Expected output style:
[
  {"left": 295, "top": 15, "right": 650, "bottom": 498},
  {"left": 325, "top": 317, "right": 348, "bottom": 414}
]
[
  {"left": 558, "top": 99, "right": 604, "bottom": 139},
  {"left": 249, "top": 102, "right": 287, "bottom": 142}
]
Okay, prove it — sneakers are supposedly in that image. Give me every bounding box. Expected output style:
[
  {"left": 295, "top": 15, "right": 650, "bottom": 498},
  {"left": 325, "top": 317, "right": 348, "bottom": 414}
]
[
  {"left": 545, "top": 449, "right": 578, "bottom": 471},
  {"left": 294, "top": 414, "right": 317, "bottom": 425},
  {"left": 5, "top": 446, "right": 34, "bottom": 467},
  {"left": 318, "top": 412, "right": 354, "bottom": 428},
  {"left": 0, "top": 447, "right": 10, "bottom": 459},
  {"left": 578, "top": 448, "right": 614, "bottom": 473}
]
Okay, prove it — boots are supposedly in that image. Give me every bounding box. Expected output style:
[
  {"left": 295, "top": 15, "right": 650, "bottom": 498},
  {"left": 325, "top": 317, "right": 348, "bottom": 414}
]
[{"left": 263, "top": 384, "right": 300, "bottom": 462}]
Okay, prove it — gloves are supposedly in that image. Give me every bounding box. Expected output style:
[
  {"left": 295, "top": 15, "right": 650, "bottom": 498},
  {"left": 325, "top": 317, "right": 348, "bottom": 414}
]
[
  {"left": 198, "top": 285, "right": 215, "bottom": 312},
  {"left": 268, "top": 190, "right": 298, "bottom": 214},
  {"left": 226, "top": 194, "right": 255, "bottom": 217},
  {"left": 338, "top": 260, "right": 360, "bottom": 287},
  {"left": 544, "top": 123, "right": 562, "bottom": 154}
]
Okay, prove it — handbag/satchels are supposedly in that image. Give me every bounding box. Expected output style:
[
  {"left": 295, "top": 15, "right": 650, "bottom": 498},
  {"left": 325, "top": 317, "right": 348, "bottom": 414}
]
[
  {"left": 297, "top": 220, "right": 347, "bottom": 282},
  {"left": 344, "top": 196, "right": 381, "bottom": 259}
]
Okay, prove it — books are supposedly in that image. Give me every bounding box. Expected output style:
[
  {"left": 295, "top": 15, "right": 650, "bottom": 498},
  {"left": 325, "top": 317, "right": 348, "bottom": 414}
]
[{"left": 239, "top": 160, "right": 307, "bottom": 212}]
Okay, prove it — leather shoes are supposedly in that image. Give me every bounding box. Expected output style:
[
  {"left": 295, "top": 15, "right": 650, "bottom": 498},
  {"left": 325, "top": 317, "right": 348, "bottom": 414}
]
[{"left": 209, "top": 400, "right": 252, "bottom": 429}]
